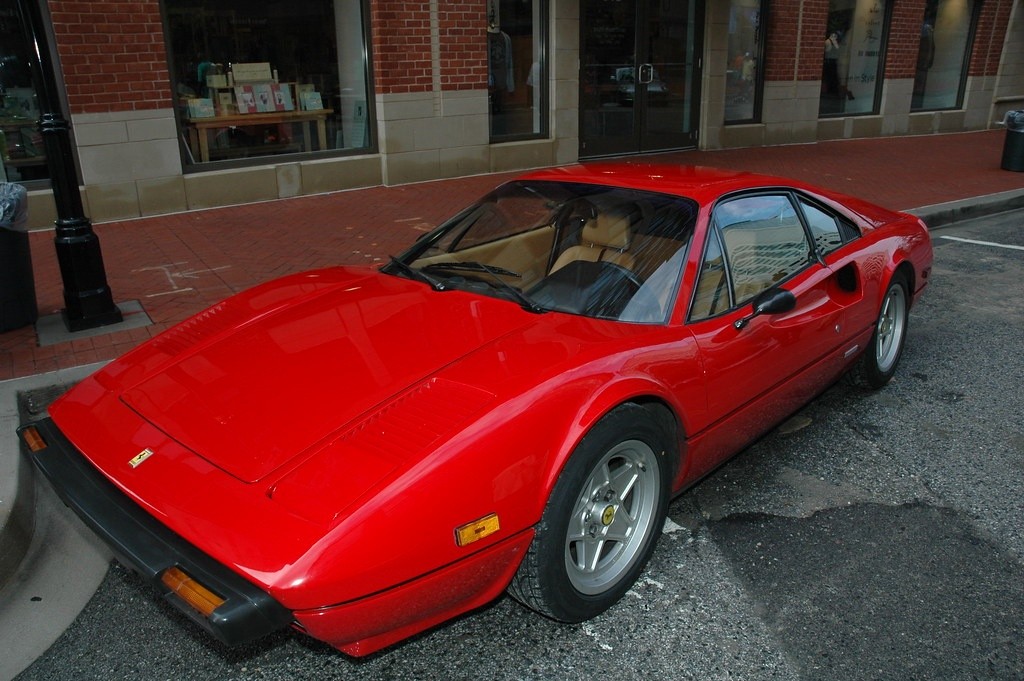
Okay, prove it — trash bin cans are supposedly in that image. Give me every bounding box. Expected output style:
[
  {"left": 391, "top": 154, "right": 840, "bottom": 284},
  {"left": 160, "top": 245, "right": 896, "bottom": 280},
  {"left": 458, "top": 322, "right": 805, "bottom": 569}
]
[
  {"left": 0, "top": 182, "right": 46, "bottom": 330},
  {"left": 1000, "top": 110, "right": 1024, "bottom": 172}
]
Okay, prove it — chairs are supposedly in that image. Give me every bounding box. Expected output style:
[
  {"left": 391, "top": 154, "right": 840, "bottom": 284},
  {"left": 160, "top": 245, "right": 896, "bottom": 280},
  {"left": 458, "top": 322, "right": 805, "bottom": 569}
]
[
  {"left": 658, "top": 229, "right": 765, "bottom": 319},
  {"left": 550, "top": 211, "right": 637, "bottom": 275}
]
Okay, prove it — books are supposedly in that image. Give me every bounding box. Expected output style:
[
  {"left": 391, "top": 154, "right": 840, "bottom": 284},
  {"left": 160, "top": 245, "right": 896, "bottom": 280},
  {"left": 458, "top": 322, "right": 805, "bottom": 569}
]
[{"left": 179, "top": 84, "right": 323, "bottom": 118}]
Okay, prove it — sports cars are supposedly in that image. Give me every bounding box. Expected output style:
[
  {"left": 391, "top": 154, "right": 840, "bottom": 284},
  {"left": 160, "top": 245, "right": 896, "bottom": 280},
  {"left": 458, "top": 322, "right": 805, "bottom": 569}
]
[{"left": 14, "top": 156, "right": 937, "bottom": 659}]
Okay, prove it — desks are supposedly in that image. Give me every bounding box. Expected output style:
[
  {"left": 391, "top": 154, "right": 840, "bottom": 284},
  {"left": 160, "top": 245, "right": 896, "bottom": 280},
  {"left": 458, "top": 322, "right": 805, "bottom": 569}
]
[{"left": 181, "top": 109, "right": 334, "bottom": 163}]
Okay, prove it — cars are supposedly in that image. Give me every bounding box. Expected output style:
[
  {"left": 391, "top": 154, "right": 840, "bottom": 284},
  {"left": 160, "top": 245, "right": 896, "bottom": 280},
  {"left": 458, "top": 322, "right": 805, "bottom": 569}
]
[{"left": 585, "top": 72, "right": 670, "bottom": 107}]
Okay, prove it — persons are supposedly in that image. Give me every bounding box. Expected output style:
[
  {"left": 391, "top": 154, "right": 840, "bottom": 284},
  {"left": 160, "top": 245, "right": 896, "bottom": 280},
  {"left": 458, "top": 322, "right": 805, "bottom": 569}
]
[
  {"left": 911, "top": 24, "right": 935, "bottom": 108},
  {"left": 733, "top": 52, "right": 753, "bottom": 101},
  {"left": 321, "top": 75, "right": 342, "bottom": 148},
  {"left": 820, "top": 34, "right": 845, "bottom": 113},
  {"left": 487, "top": 25, "right": 514, "bottom": 134},
  {"left": 197, "top": 53, "right": 217, "bottom": 96}
]
